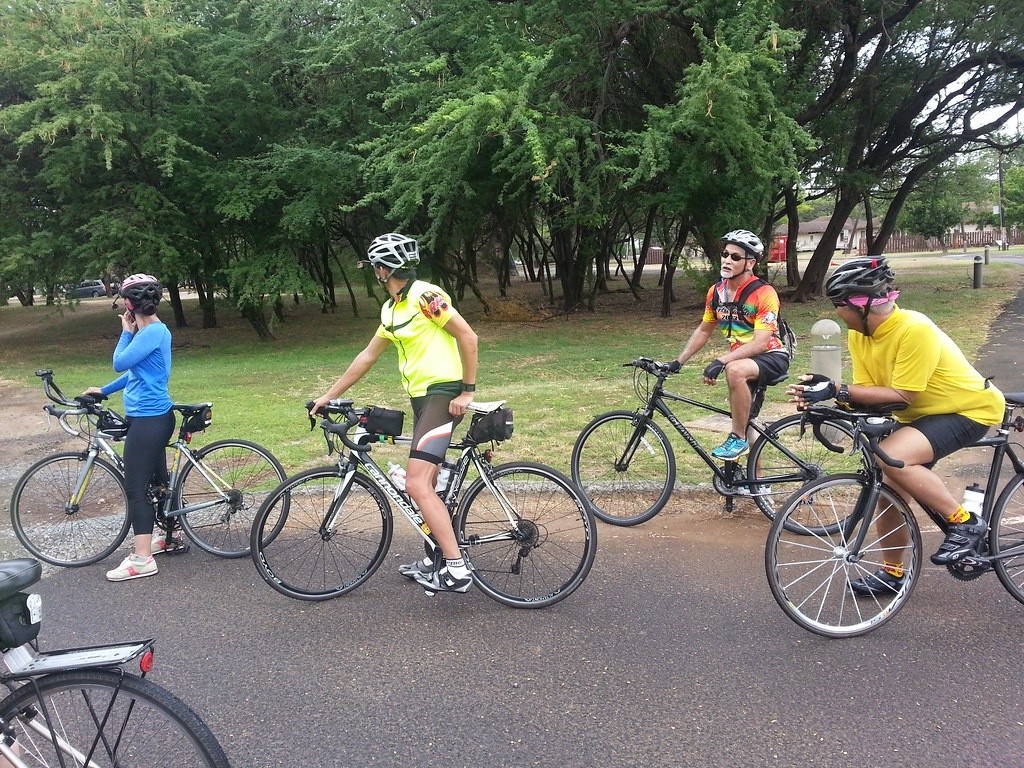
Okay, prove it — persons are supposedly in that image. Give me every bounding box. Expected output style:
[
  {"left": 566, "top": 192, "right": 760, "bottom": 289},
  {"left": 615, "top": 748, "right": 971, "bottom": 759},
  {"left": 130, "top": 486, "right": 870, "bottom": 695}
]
[
  {"left": 310, "top": 232, "right": 479, "bottom": 594},
  {"left": 663, "top": 231, "right": 790, "bottom": 515},
  {"left": 786, "top": 255, "right": 1006, "bottom": 596},
  {"left": 82, "top": 273, "right": 185, "bottom": 581}
]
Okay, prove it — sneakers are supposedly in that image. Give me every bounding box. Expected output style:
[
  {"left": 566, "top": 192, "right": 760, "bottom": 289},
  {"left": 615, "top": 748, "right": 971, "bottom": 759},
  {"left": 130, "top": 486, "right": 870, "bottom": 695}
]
[
  {"left": 399, "top": 558, "right": 434, "bottom": 578},
  {"left": 847, "top": 570, "right": 905, "bottom": 594},
  {"left": 106, "top": 552, "right": 158, "bottom": 581},
  {"left": 415, "top": 566, "right": 473, "bottom": 593},
  {"left": 745, "top": 484, "right": 771, "bottom": 493},
  {"left": 711, "top": 433, "right": 750, "bottom": 461},
  {"left": 150, "top": 529, "right": 184, "bottom": 555},
  {"left": 931, "top": 512, "right": 987, "bottom": 565}
]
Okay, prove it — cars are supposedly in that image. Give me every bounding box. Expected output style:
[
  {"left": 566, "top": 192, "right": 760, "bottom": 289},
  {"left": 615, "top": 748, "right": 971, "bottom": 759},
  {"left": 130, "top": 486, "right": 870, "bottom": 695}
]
[{"left": 65, "top": 278, "right": 121, "bottom": 300}]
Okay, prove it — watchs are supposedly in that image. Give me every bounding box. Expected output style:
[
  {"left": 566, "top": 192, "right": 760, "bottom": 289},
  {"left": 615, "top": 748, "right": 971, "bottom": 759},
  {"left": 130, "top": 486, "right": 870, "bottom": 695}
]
[{"left": 837, "top": 384, "right": 849, "bottom": 401}]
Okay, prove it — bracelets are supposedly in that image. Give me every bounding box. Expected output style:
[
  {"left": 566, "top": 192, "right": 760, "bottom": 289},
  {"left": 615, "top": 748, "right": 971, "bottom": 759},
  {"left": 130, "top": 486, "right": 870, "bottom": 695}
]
[{"left": 460, "top": 383, "right": 476, "bottom": 391}]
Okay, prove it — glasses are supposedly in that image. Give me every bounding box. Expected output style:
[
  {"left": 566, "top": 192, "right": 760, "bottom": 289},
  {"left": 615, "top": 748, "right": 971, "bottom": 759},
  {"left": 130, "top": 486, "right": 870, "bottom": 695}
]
[
  {"left": 721, "top": 249, "right": 753, "bottom": 261},
  {"left": 832, "top": 301, "right": 847, "bottom": 309}
]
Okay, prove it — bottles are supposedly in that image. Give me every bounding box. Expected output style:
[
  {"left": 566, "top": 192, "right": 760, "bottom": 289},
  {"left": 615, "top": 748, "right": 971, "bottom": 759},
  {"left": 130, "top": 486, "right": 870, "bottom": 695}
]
[
  {"left": 434, "top": 462, "right": 455, "bottom": 494},
  {"left": 386, "top": 461, "right": 425, "bottom": 522},
  {"left": 962, "top": 482, "right": 985, "bottom": 518}
]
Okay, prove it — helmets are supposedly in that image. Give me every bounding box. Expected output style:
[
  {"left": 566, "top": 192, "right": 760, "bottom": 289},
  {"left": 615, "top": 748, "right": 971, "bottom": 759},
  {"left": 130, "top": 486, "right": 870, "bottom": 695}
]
[
  {"left": 368, "top": 233, "right": 420, "bottom": 283},
  {"left": 721, "top": 229, "right": 764, "bottom": 261},
  {"left": 826, "top": 254, "right": 899, "bottom": 308},
  {"left": 119, "top": 274, "right": 163, "bottom": 315}
]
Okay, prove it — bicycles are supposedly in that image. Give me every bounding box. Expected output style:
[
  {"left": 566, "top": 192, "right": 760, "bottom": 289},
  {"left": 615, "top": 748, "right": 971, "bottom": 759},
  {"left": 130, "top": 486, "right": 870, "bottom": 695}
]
[
  {"left": 763, "top": 387, "right": 1024, "bottom": 641},
  {"left": 248, "top": 396, "right": 599, "bottom": 611},
  {"left": 569, "top": 354, "right": 881, "bottom": 538},
  {"left": 0, "top": 557, "right": 235, "bottom": 768},
  {"left": 8, "top": 367, "right": 292, "bottom": 569}
]
